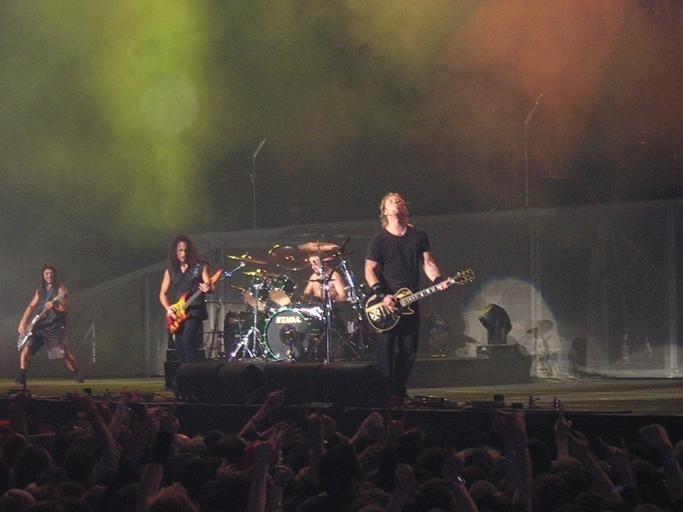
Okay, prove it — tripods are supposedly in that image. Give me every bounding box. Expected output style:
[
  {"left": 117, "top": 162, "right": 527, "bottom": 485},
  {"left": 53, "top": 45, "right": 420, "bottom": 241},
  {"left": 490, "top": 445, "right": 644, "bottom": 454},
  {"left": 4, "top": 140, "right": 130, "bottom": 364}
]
[
  {"left": 307, "top": 304, "right": 361, "bottom": 365},
  {"left": 228, "top": 283, "right": 271, "bottom": 363}
]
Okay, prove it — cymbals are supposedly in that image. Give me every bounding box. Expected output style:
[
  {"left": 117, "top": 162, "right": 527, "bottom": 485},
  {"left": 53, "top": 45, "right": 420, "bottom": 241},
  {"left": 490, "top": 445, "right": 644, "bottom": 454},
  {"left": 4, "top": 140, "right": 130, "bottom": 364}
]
[
  {"left": 323, "top": 249, "right": 357, "bottom": 262},
  {"left": 310, "top": 278, "right": 336, "bottom": 284},
  {"left": 227, "top": 254, "right": 266, "bottom": 264},
  {"left": 244, "top": 270, "right": 278, "bottom": 278},
  {"left": 270, "top": 242, "right": 310, "bottom": 270},
  {"left": 298, "top": 241, "right": 337, "bottom": 252}
]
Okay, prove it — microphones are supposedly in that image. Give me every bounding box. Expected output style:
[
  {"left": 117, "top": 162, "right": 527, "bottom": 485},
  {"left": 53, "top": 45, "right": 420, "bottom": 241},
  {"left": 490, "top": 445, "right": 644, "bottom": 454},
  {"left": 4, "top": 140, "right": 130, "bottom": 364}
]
[
  {"left": 285, "top": 331, "right": 293, "bottom": 345},
  {"left": 338, "top": 236, "right": 351, "bottom": 254}
]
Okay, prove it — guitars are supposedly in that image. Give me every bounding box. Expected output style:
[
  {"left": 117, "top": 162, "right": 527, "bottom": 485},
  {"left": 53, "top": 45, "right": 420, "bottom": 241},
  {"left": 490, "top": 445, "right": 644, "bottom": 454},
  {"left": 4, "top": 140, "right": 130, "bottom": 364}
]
[
  {"left": 167, "top": 267, "right": 225, "bottom": 334},
  {"left": 15, "top": 291, "right": 66, "bottom": 351},
  {"left": 363, "top": 269, "right": 475, "bottom": 332}
]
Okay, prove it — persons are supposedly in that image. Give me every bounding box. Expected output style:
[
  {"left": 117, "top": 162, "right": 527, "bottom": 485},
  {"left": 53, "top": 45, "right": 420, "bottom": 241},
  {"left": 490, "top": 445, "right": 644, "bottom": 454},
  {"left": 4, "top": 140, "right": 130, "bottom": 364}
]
[
  {"left": 364, "top": 191, "right": 451, "bottom": 385},
  {"left": 1, "top": 386, "right": 683, "bottom": 512},
  {"left": 14, "top": 264, "right": 84, "bottom": 385},
  {"left": 304, "top": 253, "right": 348, "bottom": 302},
  {"left": 159, "top": 236, "right": 217, "bottom": 362}
]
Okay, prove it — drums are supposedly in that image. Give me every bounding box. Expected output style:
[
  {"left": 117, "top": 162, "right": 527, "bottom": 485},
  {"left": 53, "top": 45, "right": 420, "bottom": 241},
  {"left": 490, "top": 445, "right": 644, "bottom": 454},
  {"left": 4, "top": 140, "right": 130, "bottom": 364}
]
[
  {"left": 262, "top": 302, "right": 327, "bottom": 360},
  {"left": 242, "top": 279, "right": 269, "bottom": 312},
  {"left": 225, "top": 310, "right": 267, "bottom": 360},
  {"left": 268, "top": 274, "right": 298, "bottom": 305}
]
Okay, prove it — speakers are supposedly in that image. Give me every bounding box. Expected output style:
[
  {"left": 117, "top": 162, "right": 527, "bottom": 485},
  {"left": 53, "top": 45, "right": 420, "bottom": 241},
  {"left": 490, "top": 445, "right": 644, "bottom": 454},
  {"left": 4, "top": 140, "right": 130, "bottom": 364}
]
[{"left": 173, "top": 360, "right": 383, "bottom": 404}]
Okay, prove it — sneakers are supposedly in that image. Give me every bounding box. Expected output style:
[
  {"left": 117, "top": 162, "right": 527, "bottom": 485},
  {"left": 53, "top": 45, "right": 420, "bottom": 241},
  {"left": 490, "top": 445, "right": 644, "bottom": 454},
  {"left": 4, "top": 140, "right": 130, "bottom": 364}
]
[
  {"left": 15, "top": 374, "right": 26, "bottom": 386},
  {"left": 72, "top": 373, "right": 85, "bottom": 383}
]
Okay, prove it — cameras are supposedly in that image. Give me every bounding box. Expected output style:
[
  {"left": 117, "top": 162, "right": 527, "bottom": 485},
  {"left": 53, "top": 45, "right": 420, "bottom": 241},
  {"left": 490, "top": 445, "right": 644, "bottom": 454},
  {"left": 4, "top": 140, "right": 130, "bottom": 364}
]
[{"left": 84, "top": 388, "right": 91, "bottom": 397}]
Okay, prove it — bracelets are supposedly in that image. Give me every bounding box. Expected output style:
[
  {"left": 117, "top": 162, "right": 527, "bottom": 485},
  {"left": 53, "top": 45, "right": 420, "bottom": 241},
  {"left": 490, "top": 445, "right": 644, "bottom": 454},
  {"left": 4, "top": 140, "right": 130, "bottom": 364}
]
[{"left": 434, "top": 277, "right": 445, "bottom": 283}]
